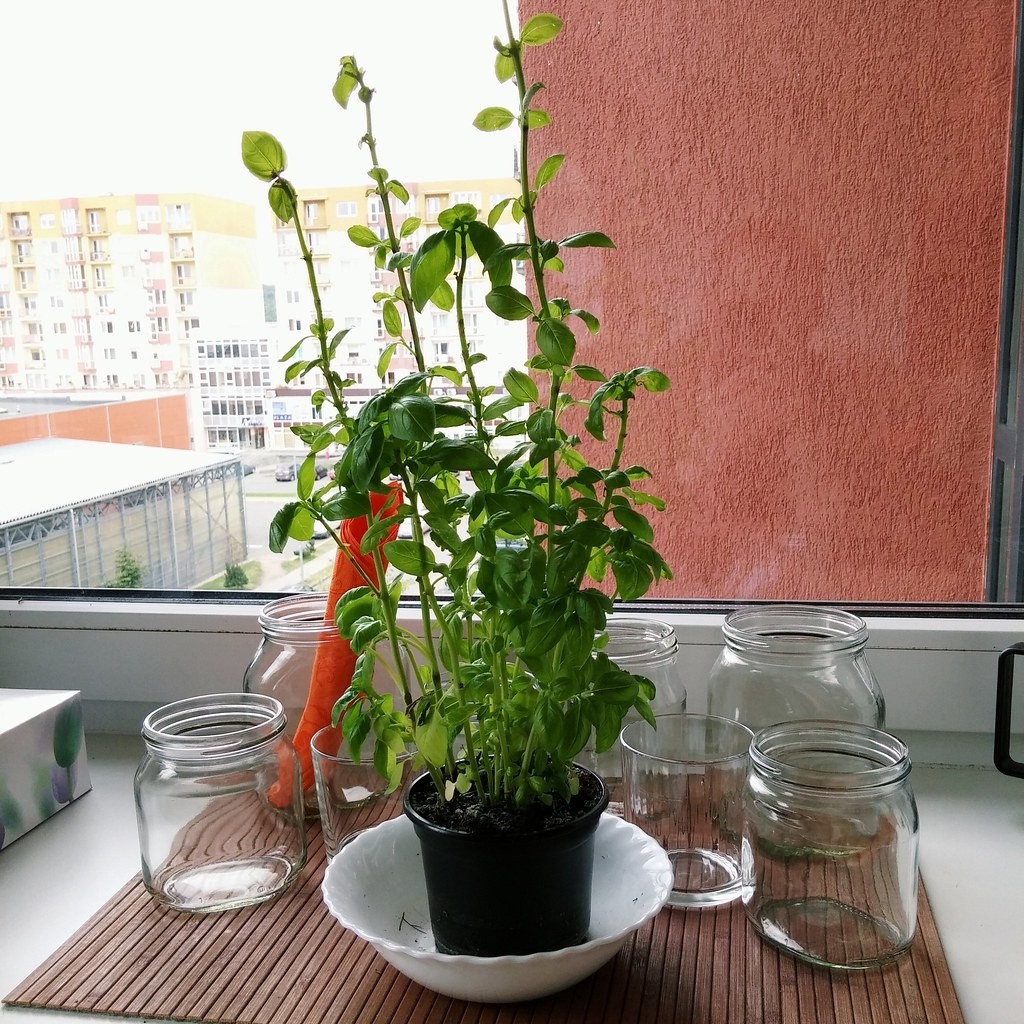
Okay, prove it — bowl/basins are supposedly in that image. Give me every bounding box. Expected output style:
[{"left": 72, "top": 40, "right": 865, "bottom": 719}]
[{"left": 320, "top": 814, "right": 675, "bottom": 1003}]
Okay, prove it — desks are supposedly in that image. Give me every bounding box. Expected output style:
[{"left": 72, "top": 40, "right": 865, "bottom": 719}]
[{"left": 1, "top": 731, "right": 965, "bottom": 1024}]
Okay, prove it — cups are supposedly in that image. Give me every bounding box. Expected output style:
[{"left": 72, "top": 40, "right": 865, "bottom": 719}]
[
  {"left": 619, "top": 710, "right": 749, "bottom": 907},
  {"left": 312, "top": 723, "right": 419, "bottom": 870}
]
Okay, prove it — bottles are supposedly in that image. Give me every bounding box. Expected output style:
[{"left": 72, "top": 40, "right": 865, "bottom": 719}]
[
  {"left": 574, "top": 619, "right": 684, "bottom": 818},
  {"left": 133, "top": 692, "right": 309, "bottom": 913},
  {"left": 739, "top": 720, "right": 921, "bottom": 967},
  {"left": 240, "top": 593, "right": 394, "bottom": 820},
  {"left": 707, "top": 605, "right": 887, "bottom": 858}
]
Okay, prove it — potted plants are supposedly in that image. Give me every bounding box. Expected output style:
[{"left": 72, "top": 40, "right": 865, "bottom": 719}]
[{"left": 240, "top": 2, "right": 686, "bottom": 959}]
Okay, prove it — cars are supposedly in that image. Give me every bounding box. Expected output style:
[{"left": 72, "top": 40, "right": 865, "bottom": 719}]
[
  {"left": 328, "top": 469, "right": 335, "bottom": 481},
  {"left": 314, "top": 464, "right": 328, "bottom": 480},
  {"left": 275, "top": 464, "right": 300, "bottom": 481},
  {"left": 227, "top": 464, "right": 255, "bottom": 476},
  {"left": 397, "top": 511, "right": 432, "bottom": 539},
  {"left": 311, "top": 518, "right": 340, "bottom": 539},
  {"left": 495, "top": 539, "right": 529, "bottom": 551},
  {"left": 389, "top": 473, "right": 402, "bottom": 481}
]
